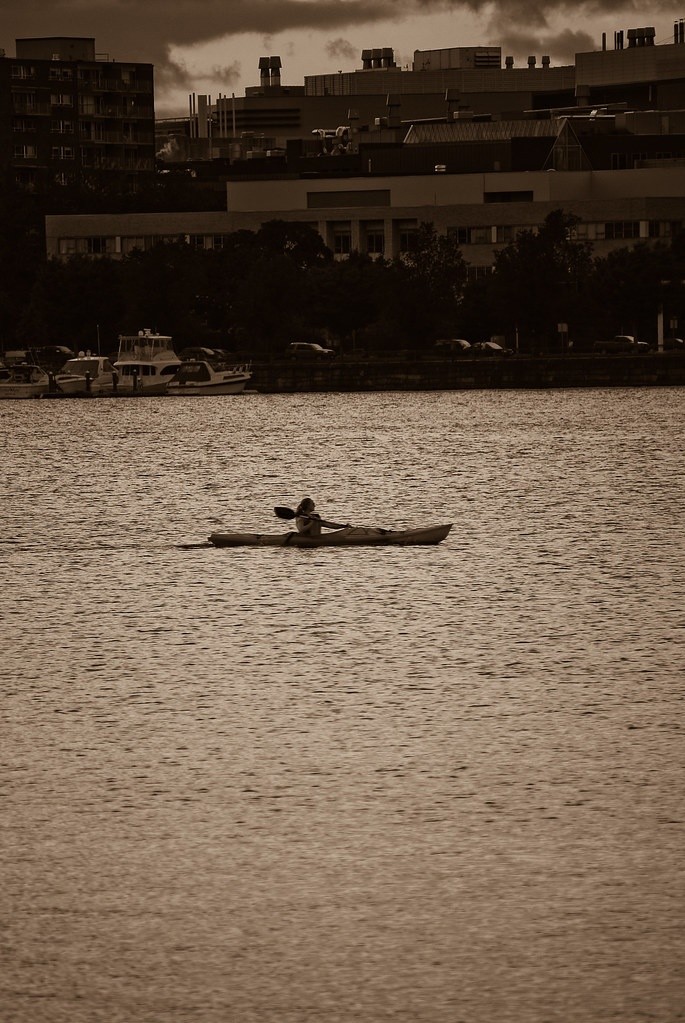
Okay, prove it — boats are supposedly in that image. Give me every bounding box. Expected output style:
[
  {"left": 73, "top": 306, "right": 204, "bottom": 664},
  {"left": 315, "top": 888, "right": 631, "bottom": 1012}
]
[
  {"left": 206, "top": 522, "right": 455, "bottom": 548},
  {"left": 0, "top": 324, "right": 253, "bottom": 400}
]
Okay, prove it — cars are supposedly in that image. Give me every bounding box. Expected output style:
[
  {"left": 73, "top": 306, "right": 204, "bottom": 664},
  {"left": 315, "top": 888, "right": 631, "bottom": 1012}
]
[
  {"left": 474, "top": 341, "right": 514, "bottom": 357},
  {"left": 180, "top": 347, "right": 224, "bottom": 362}
]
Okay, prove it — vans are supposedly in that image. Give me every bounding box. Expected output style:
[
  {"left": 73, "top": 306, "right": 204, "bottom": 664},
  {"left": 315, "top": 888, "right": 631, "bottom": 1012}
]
[{"left": 434, "top": 339, "right": 471, "bottom": 355}]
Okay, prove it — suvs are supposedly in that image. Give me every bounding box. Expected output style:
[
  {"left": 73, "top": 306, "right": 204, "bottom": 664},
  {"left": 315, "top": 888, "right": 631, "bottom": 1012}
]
[{"left": 284, "top": 342, "right": 337, "bottom": 362}]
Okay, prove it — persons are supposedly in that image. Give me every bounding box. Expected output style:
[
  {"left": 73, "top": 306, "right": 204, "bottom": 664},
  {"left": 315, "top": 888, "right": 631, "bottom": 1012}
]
[{"left": 295, "top": 498, "right": 322, "bottom": 532}]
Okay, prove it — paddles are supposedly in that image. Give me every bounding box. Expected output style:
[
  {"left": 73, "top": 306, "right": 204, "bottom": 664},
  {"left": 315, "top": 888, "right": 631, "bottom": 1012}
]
[{"left": 273, "top": 506, "right": 349, "bottom": 528}]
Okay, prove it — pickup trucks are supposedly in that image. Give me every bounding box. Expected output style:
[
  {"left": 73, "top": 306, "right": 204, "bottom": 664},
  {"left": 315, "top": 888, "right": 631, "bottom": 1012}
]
[{"left": 594, "top": 335, "right": 650, "bottom": 354}]
